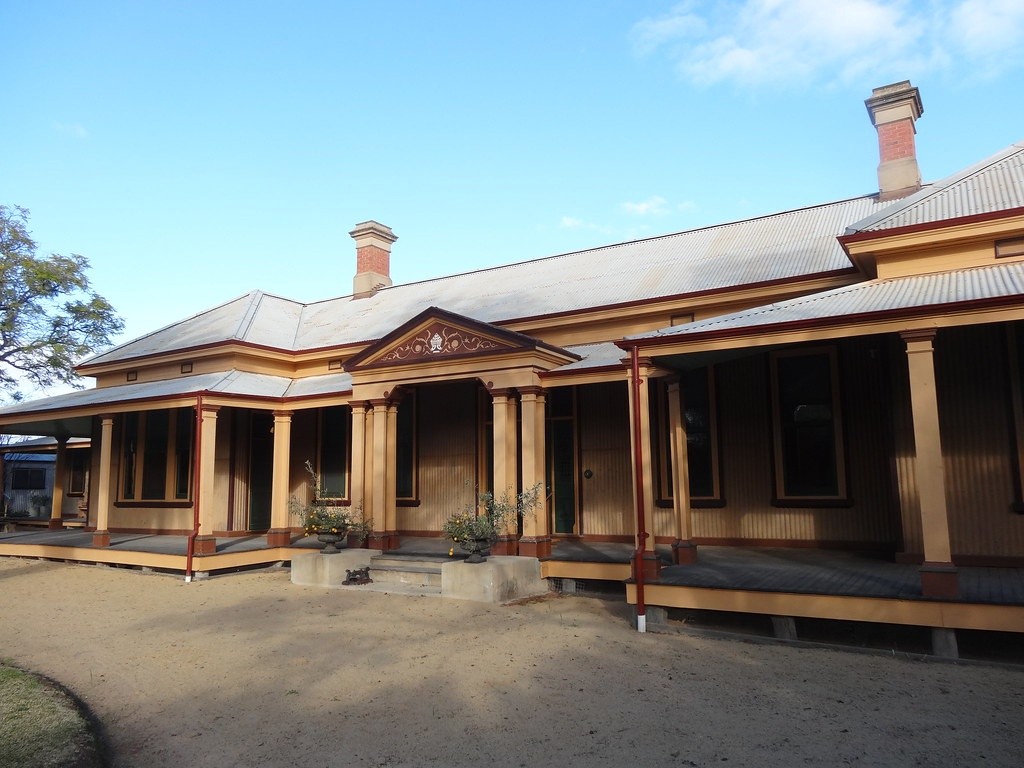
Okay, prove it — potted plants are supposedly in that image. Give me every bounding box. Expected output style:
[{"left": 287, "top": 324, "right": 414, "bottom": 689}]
[
  {"left": 39, "top": 495, "right": 52, "bottom": 516},
  {"left": 29, "top": 496, "right": 40, "bottom": 517}
]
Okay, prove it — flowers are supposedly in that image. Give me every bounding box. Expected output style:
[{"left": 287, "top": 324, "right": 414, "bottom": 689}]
[
  {"left": 440, "top": 481, "right": 543, "bottom": 556},
  {"left": 287, "top": 459, "right": 381, "bottom": 542}
]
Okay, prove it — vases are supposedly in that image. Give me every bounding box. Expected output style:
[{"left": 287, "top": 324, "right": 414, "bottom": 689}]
[
  {"left": 316, "top": 529, "right": 344, "bottom": 553},
  {"left": 460, "top": 538, "right": 490, "bottom": 564}
]
